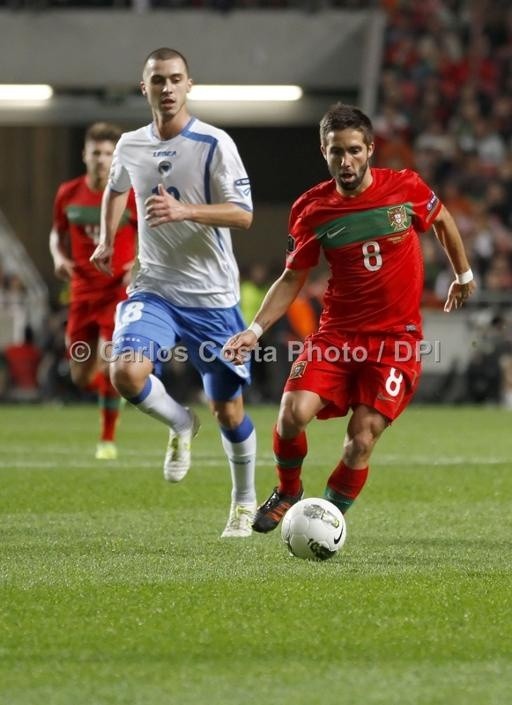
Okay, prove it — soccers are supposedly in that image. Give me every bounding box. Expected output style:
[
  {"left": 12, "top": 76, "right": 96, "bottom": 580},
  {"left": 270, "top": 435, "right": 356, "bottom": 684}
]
[{"left": 280, "top": 498, "right": 347, "bottom": 562}]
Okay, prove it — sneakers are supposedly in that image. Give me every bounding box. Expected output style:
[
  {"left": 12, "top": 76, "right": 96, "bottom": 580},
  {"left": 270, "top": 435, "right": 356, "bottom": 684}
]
[
  {"left": 163, "top": 407, "right": 201, "bottom": 484},
  {"left": 95, "top": 441, "right": 116, "bottom": 460},
  {"left": 253, "top": 480, "right": 305, "bottom": 533},
  {"left": 220, "top": 502, "right": 255, "bottom": 537}
]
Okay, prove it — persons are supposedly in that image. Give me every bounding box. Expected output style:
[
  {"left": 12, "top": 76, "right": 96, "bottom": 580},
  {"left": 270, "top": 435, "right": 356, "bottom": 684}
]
[
  {"left": 88, "top": 48, "right": 265, "bottom": 541},
  {"left": 46, "top": 121, "right": 140, "bottom": 463},
  {"left": 218, "top": 99, "right": 480, "bottom": 537},
  {"left": 0, "top": 252, "right": 335, "bottom": 411},
  {"left": 368, "top": 0, "right": 512, "bottom": 407}
]
